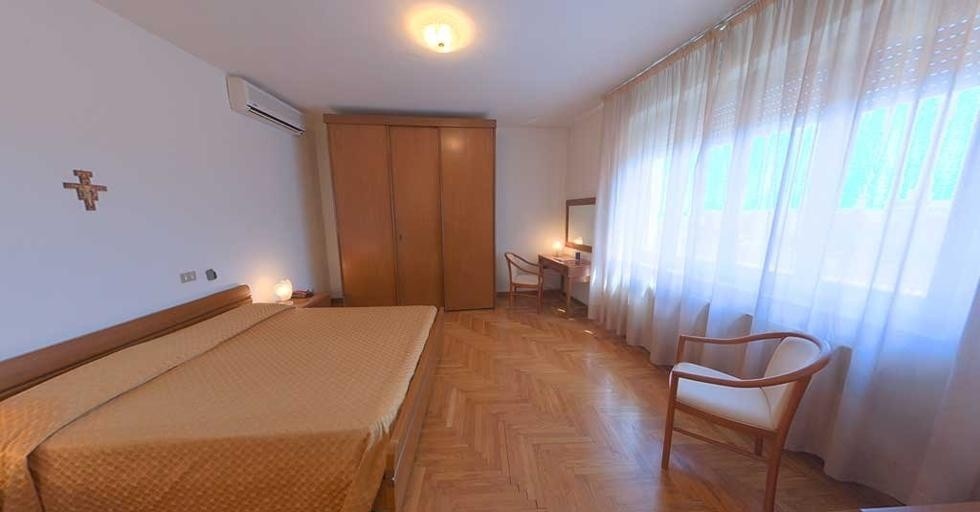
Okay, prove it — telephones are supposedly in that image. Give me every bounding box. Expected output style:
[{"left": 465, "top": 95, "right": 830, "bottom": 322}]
[{"left": 292, "top": 289, "right": 315, "bottom": 298}]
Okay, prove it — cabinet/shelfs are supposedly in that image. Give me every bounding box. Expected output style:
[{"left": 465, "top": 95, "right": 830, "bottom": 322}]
[{"left": 322, "top": 107, "right": 498, "bottom": 313}]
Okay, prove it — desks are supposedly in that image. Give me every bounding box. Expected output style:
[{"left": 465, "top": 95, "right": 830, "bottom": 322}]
[{"left": 534, "top": 253, "right": 595, "bottom": 320}]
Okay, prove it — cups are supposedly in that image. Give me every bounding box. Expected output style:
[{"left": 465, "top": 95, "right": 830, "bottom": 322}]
[{"left": 575, "top": 252, "right": 580, "bottom": 259}]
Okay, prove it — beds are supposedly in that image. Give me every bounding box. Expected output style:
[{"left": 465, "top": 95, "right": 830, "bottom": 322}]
[{"left": 1, "top": 280, "right": 450, "bottom": 510}]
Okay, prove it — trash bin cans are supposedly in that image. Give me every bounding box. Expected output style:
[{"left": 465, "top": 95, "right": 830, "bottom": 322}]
[{"left": 552, "top": 303, "right": 568, "bottom": 317}]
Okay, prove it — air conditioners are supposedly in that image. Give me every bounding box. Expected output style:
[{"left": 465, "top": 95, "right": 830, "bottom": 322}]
[{"left": 222, "top": 71, "right": 310, "bottom": 139}]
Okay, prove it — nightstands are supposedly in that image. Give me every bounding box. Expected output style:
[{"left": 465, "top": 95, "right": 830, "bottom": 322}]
[{"left": 276, "top": 289, "right": 334, "bottom": 307}]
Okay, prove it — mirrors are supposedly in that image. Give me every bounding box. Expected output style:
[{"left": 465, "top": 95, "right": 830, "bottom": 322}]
[{"left": 565, "top": 197, "right": 598, "bottom": 253}]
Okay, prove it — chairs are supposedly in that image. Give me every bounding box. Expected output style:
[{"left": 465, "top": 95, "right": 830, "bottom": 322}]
[
  {"left": 505, "top": 248, "right": 549, "bottom": 317},
  {"left": 660, "top": 329, "right": 832, "bottom": 512}
]
